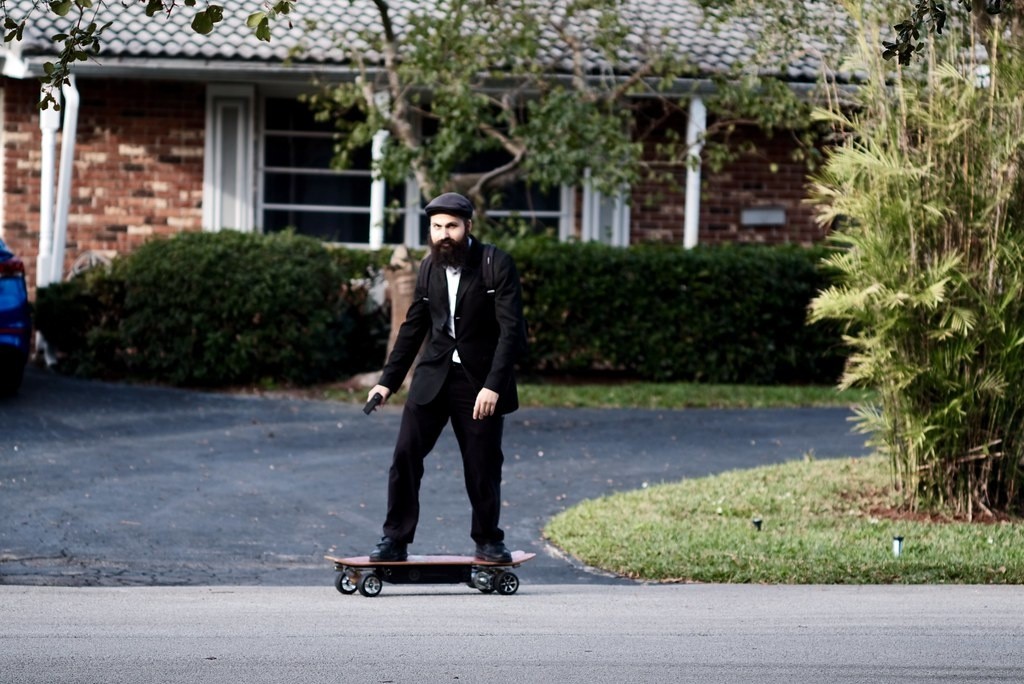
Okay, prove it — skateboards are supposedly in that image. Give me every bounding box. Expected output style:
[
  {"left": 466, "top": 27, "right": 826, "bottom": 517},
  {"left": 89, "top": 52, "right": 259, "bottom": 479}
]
[{"left": 323, "top": 548, "right": 537, "bottom": 597}]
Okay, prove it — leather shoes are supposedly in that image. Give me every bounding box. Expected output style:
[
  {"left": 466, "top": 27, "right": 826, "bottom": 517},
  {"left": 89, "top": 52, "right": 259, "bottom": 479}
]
[
  {"left": 476, "top": 541, "right": 512, "bottom": 562},
  {"left": 370, "top": 536, "right": 407, "bottom": 561}
]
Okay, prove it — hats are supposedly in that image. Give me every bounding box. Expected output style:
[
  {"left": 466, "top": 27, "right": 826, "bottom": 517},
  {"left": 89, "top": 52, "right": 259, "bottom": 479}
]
[{"left": 425, "top": 192, "right": 473, "bottom": 219}]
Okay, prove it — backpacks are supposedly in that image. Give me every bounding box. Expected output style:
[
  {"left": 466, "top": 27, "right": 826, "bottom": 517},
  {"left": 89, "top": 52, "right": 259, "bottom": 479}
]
[{"left": 418, "top": 244, "right": 532, "bottom": 375}]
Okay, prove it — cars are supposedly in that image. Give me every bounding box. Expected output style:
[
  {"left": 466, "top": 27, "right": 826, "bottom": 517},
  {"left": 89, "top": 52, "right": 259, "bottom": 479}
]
[{"left": 1, "top": 238, "right": 29, "bottom": 390}]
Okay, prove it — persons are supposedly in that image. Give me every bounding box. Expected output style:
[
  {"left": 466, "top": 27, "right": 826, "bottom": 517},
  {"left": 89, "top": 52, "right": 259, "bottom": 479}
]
[{"left": 363, "top": 190, "right": 524, "bottom": 564}]
